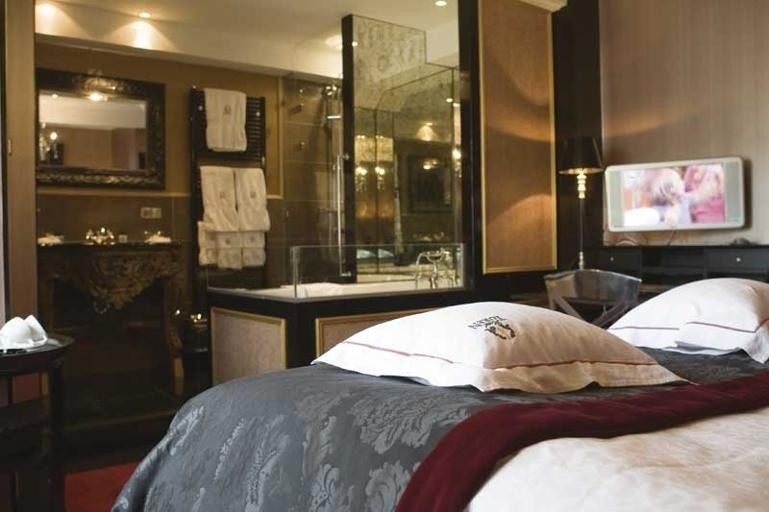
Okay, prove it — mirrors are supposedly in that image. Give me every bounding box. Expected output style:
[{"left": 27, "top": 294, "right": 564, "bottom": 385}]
[{"left": 35, "top": 67, "right": 168, "bottom": 192}]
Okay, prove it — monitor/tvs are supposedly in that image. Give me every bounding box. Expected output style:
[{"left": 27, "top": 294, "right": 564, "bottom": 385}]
[{"left": 605, "top": 156, "right": 745, "bottom": 232}]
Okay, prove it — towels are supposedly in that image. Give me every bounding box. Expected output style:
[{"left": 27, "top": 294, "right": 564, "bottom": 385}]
[{"left": 197, "top": 86, "right": 271, "bottom": 271}]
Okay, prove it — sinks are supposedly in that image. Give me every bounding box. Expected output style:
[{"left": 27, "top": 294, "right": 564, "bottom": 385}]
[{"left": 255, "top": 281, "right": 453, "bottom": 299}]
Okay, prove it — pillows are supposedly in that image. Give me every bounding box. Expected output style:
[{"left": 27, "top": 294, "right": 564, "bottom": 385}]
[
  {"left": 607, "top": 278, "right": 769, "bottom": 365},
  {"left": 310, "top": 300, "right": 702, "bottom": 395}
]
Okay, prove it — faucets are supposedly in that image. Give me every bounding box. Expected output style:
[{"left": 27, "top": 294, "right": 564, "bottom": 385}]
[
  {"left": 411, "top": 246, "right": 453, "bottom": 288},
  {"left": 84, "top": 228, "right": 115, "bottom": 245}
]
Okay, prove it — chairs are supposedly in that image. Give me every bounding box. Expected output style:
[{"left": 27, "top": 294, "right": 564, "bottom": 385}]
[{"left": 542, "top": 269, "right": 643, "bottom": 328}]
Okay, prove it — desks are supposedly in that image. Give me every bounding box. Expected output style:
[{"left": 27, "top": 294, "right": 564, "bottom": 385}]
[{"left": 1, "top": 331, "right": 77, "bottom": 511}]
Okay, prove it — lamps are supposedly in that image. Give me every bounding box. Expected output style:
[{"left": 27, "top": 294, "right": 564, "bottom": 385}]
[{"left": 557, "top": 132, "right": 606, "bottom": 269}]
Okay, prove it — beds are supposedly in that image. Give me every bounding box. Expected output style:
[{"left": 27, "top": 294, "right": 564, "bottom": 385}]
[{"left": 111, "top": 346, "right": 769, "bottom": 510}]
